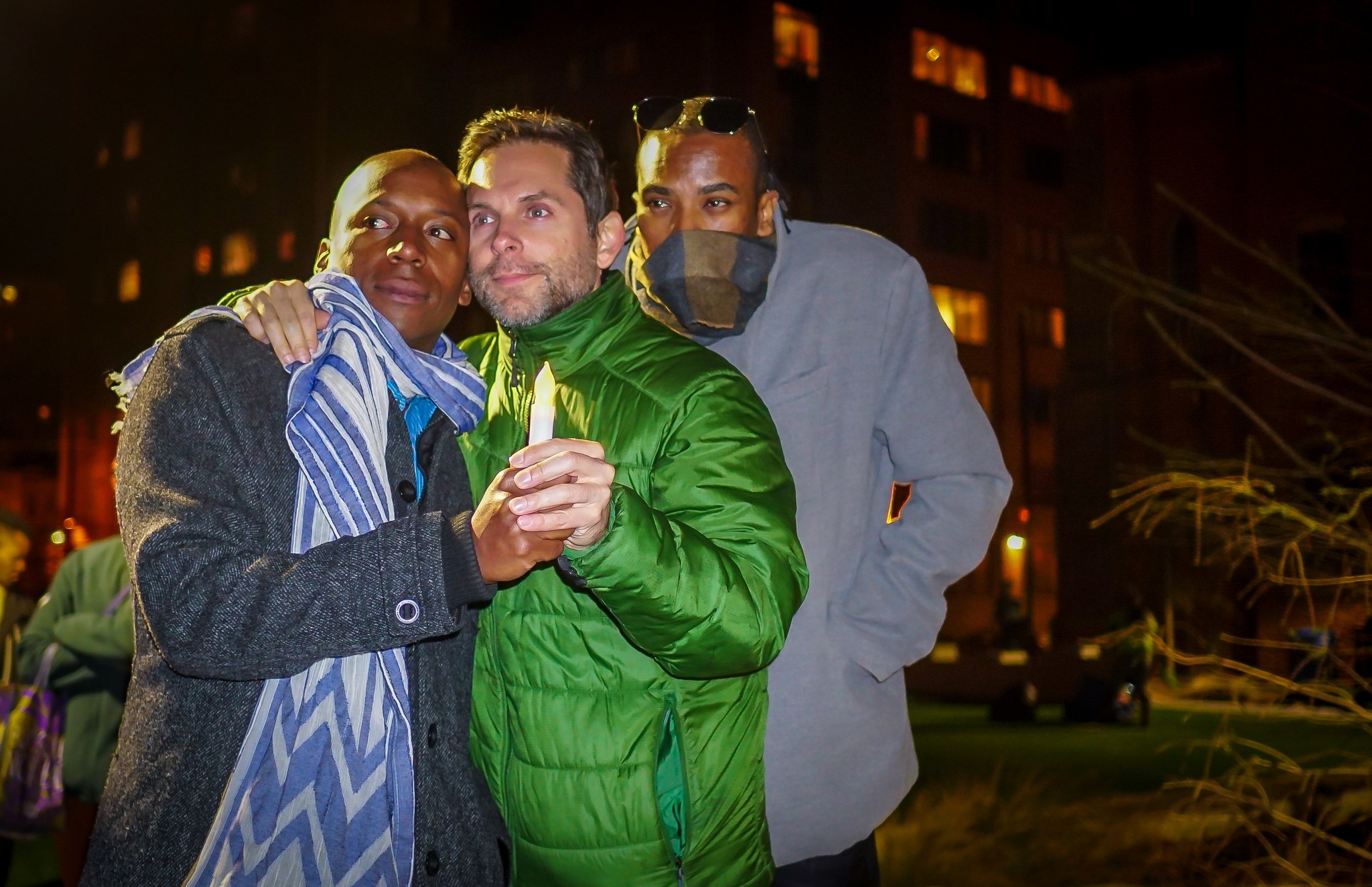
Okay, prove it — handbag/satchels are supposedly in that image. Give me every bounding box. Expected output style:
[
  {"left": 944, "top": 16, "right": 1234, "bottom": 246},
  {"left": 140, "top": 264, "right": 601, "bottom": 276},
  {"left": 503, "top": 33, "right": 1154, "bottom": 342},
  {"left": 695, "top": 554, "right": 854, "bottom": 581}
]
[{"left": 0, "top": 643, "right": 67, "bottom": 834}]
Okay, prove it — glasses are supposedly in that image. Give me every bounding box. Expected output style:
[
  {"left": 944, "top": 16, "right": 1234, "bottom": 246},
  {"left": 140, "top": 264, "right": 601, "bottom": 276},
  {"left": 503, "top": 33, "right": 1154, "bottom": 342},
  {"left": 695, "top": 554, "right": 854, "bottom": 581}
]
[{"left": 633, "top": 93, "right": 771, "bottom": 158}]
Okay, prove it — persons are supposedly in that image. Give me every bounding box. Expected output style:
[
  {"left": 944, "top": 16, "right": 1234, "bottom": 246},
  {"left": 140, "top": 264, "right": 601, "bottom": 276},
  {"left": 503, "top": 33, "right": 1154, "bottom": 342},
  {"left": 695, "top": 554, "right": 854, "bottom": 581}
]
[
  {"left": 608, "top": 96, "right": 1013, "bottom": 887},
  {"left": 216, "top": 103, "right": 811, "bottom": 887},
  {"left": 1, "top": 515, "right": 137, "bottom": 887},
  {"left": 80, "top": 150, "right": 577, "bottom": 887}
]
